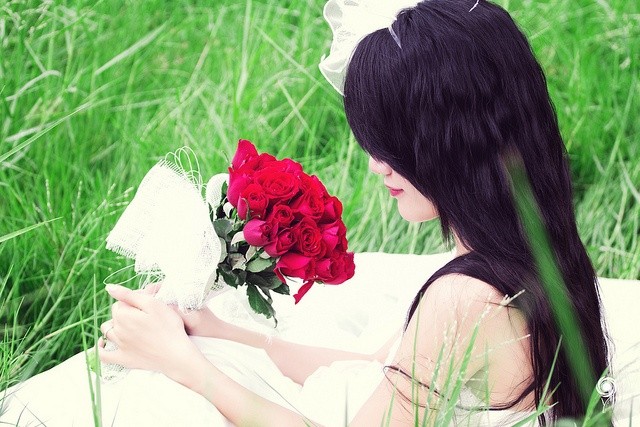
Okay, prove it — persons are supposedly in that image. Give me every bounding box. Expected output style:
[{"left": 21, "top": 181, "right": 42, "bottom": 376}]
[{"left": 95, "top": 0, "right": 616, "bottom": 427}]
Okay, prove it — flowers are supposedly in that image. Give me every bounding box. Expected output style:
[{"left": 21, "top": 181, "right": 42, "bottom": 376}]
[{"left": 210, "top": 137, "right": 359, "bottom": 331}]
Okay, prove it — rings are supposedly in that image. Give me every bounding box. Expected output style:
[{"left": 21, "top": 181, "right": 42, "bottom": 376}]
[{"left": 104, "top": 327, "right": 113, "bottom": 340}]
